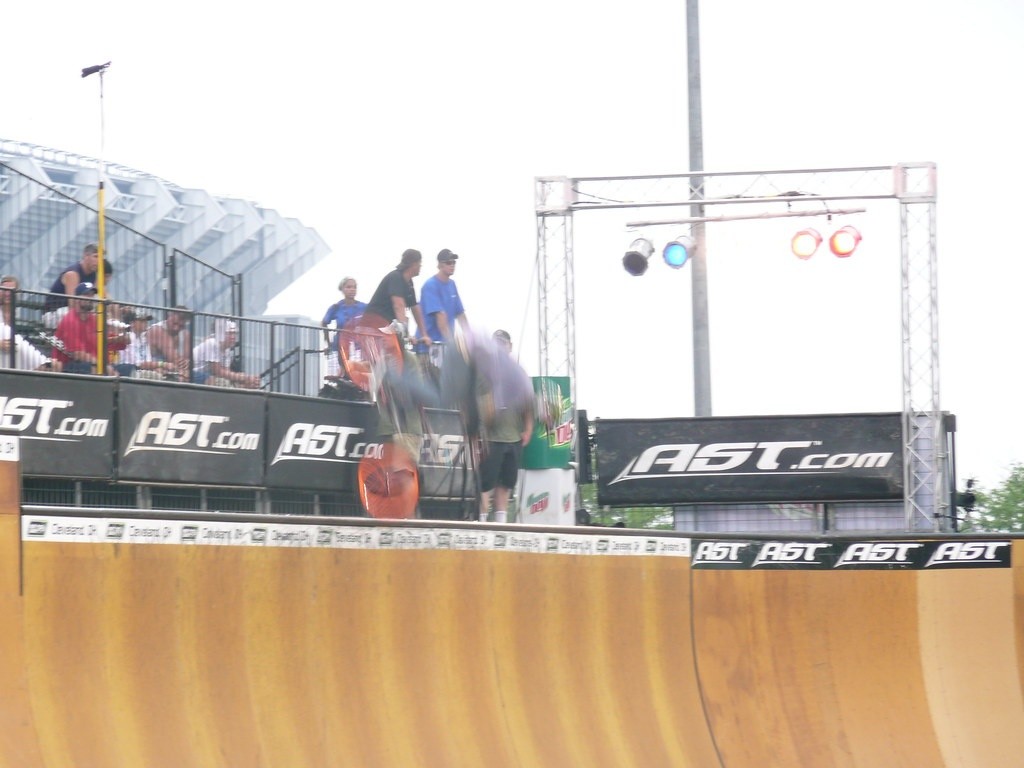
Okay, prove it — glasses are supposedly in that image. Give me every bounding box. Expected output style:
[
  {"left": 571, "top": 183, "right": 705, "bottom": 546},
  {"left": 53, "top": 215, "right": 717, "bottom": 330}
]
[{"left": 442, "top": 260, "right": 456, "bottom": 265}]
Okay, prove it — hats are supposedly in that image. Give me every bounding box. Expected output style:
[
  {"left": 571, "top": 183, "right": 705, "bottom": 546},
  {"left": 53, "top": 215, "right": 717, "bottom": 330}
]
[
  {"left": 395, "top": 249, "right": 422, "bottom": 271},
  {"left": 438, "top": 249, "right": 458, "bottom": 261},
  {"left": 492, "top": 329, "right": 511, "bottom": 344},
  {"left": 129, "top": 311, "right": 153, "bottom": 321},
  {"left": 76, "top": 282, "right": 97, "bottom": 295}
]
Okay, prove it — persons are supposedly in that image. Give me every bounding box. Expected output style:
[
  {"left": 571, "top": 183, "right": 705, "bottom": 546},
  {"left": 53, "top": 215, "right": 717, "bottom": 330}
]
[
  {"left": 321, "top": 277, "right": 367, "bottom": 371},
  {"left": 193, "top": 320, "right": 259, "bottom": 390},
  {"left": 40, "top": 244, "right": 213, "bottom": 385},
  {"left": 358, "top": 249, "right": 535, "bottom": 523},
  {"left": 0, "top": 274, "right": 63, "bottom": 372}
]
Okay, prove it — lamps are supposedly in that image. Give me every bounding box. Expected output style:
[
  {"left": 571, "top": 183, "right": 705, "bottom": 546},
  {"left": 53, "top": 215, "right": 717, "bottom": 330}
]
[
  {"left": 662, "top": 235, "right": 697, "bottom": 269},
  {"left": 829, "top": 226, "right": 862, "bottom": 258},
  {"left": 791, "top": 228, "right": 822, "bottom": 259},
  {"left": 622, "top": 237, "right": 655, "bottom": 277}
]
